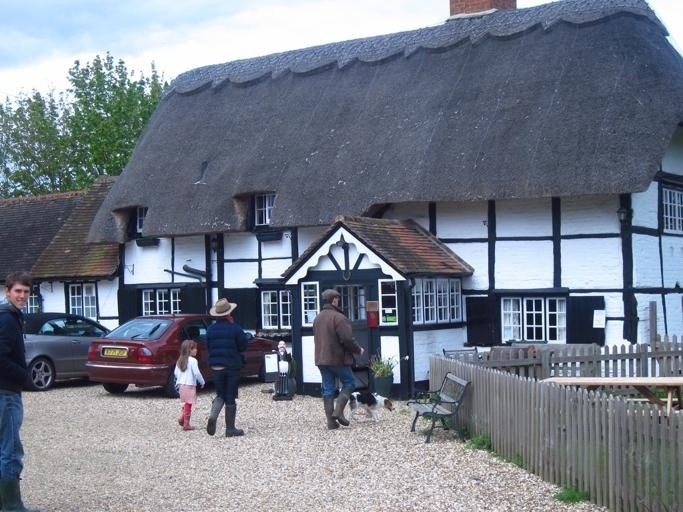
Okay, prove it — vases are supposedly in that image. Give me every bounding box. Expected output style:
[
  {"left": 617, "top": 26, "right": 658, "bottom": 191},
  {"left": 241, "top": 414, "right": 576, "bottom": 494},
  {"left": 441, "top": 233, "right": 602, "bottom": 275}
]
[{"left": 368, "top": 373, "right": 394, "bottom": 400}]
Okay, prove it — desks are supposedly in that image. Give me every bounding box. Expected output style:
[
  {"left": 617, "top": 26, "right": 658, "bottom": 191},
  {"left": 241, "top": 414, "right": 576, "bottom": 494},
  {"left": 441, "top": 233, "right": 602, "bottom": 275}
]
[{"left": 538, "top": 375, "right": 682, "bottom": 421}]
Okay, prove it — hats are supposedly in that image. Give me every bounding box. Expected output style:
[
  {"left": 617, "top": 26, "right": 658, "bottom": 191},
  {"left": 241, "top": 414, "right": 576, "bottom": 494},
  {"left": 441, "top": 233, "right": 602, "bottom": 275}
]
[
  {"left": 321, "top": 289, "right": 341, "bottom": 300},
  {"left": 209, "top": 297, "right": 238, "bottom": 317}
]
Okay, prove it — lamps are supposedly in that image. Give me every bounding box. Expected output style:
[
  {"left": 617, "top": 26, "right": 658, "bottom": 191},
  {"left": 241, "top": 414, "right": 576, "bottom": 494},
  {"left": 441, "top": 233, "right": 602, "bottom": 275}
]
[
  {"left": 616, "top": 203, "right": 629, "bottom": 222},
  {"left": 211, "top": 235, "right": 219, "bottom": 255}
]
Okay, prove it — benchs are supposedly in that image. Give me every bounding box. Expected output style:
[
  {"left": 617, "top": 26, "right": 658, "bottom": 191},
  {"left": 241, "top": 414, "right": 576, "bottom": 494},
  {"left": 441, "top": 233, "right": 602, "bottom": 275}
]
[
  {"left": 581, "top": 396, "right": 681, "bottom": 404},
  {"left": 441, "top": 346, "right": 478, "bottom": 360},
  {"left": 600, "top": 407, "right": 678, "bottom": 419},
  {"left": 406, "top": 370, "right": 471, "bottom": 444}
]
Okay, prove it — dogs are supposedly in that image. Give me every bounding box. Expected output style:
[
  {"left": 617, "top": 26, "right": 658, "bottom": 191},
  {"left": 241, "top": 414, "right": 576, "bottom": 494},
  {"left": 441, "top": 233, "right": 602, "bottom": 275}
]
[{"left": 338, "top": 390, "right": 395, "bottom": 423}]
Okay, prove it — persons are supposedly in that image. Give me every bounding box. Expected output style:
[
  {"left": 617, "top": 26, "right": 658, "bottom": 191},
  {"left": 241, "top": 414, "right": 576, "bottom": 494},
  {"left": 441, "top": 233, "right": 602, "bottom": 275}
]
[
  {"left": 205, "top": 298, "right": 248, "bottom": 438},
  {"left": 0, "top": 271, "right": 33, "bottom": 512},
  {"left": 174, "top": 340, "right": 206, "bottom": 430},
  {"left": 272, "top": 340, "right": 291, "bottom": 361},
  {"left": 312, "top": 289, "right": 365, "bottom": 429}
]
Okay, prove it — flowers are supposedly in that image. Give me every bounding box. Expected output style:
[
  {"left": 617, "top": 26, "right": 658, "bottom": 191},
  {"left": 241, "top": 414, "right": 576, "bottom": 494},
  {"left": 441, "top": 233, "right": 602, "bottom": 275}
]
[{"left": 367, "top": 349, "right": 412, "bottom": 379}]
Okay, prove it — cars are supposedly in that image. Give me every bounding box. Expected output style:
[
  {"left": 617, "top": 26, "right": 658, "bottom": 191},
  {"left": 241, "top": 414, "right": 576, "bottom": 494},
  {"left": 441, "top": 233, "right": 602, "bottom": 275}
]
[
  {"left": 21, "top": 313, "right": 111, "bottom": 391},
  {"left": 84, "top": 312, "right": 279, "bottom": 398}
]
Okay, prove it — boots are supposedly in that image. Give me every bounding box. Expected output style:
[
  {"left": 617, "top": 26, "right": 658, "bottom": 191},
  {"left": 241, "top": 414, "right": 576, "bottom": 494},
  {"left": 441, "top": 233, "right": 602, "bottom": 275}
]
[
  {"left": 183, "top": 415, "right": 195, "bottom": 430},
  {"left": 206, "top": 398, "right": 224, "bottom": 435},
  {"left": 332, "top": 389, "right": 350, "bottom": 425},
  {"left": 0, "top": 479, "right": 40, "bottom": 512},
  {"left": 324, "top": 396, "right": 339, "bottom": 429},
  {"left": 178, "top": 409, "right": 184, "bottom": 426},
  {"left": 225, "top": 404, "right": 244, "bottom": 437}
]
